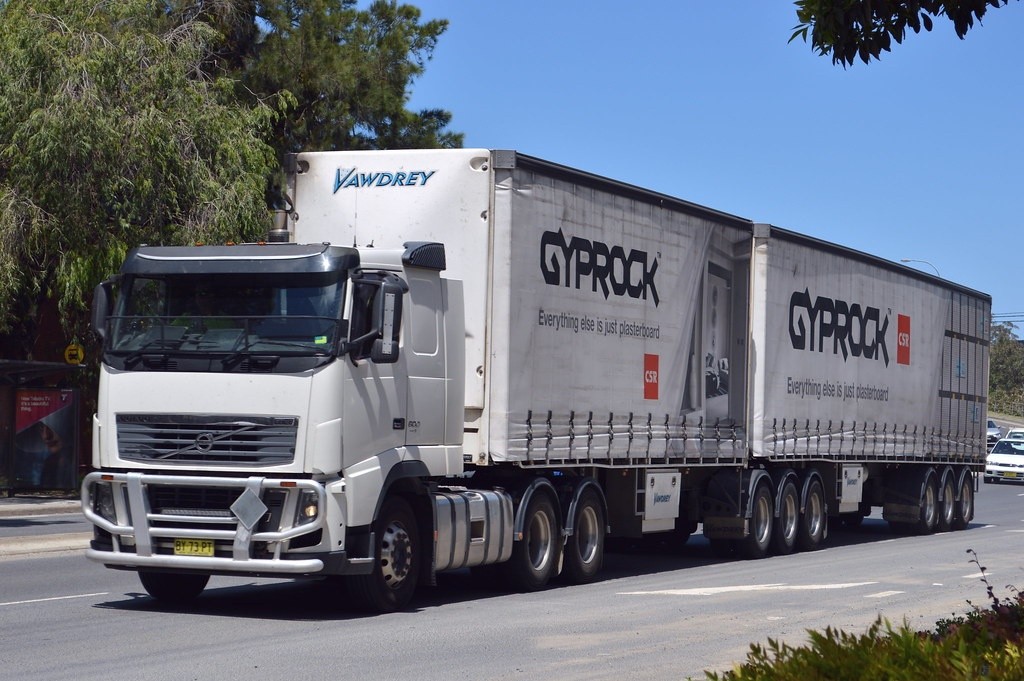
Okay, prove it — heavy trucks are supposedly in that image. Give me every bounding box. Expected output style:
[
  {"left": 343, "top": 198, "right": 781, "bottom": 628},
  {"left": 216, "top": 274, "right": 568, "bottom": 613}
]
[{"left": 80, "top": 147, "right": 992, "bottom": 589}]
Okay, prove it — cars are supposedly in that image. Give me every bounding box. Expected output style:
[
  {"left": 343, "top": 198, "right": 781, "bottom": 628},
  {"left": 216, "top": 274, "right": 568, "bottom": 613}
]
[
  {"left": 987, "top": 419, "right": 1001, "bottom": 441},
  {"left": 983, "top": 438, "right": 1024, "bottom": 484}
]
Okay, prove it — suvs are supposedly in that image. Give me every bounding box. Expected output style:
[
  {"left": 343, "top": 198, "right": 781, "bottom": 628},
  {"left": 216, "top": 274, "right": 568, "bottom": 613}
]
[{"left": 1005, "top": 426, "right": 1024, "bottom": 440}]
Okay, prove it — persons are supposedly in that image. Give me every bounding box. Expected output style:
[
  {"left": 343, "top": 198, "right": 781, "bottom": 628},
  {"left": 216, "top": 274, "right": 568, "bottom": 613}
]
[{"left": 38, "top": 424, "right": 73, "bottom": 491}]
